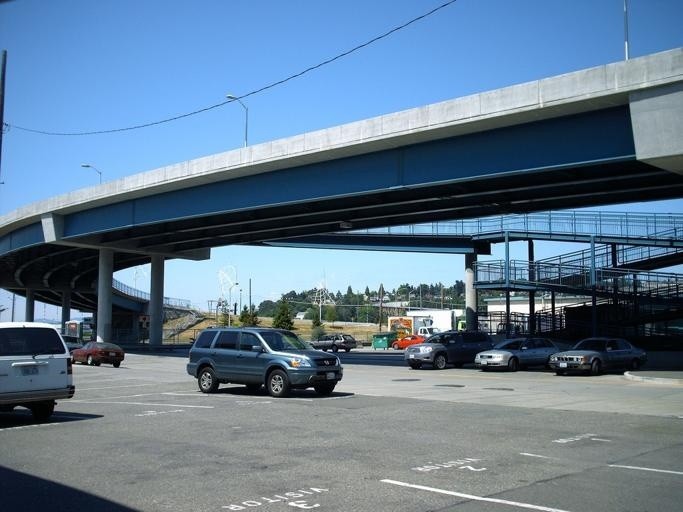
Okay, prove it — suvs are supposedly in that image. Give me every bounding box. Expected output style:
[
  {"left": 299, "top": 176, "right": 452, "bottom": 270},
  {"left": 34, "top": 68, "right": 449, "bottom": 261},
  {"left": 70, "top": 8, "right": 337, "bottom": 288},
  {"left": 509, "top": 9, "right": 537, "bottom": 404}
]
[
  {"left": 0, "top": 322, "right": 74, "bottom": 420},
  {"left": 185, "top": 325, "right": 343, "bottom": 400},
  {"left": 402, "top": 329, "right": 495, "bottom": 372},
  {"left": 310, "top": 333, "right": 356, "bottom": 355}
]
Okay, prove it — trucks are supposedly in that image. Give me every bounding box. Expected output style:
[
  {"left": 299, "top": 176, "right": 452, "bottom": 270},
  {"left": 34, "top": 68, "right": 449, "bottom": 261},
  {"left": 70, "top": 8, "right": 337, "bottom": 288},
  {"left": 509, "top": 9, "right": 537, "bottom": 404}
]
[{"left": 386, "top": 315, "right": 441, "bottom": 339}]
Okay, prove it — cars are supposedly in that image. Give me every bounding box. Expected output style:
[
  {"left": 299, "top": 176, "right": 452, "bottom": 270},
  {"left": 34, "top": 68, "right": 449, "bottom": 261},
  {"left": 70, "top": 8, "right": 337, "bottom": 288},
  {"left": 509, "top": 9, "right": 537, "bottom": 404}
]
[
  {"left": 62, "top": 334, "right": 124, "bottom": 369},
  {"left": 547, "top": 337, "right": 647, "bottom": 380},
  {"left": 474, "top": 336, "right": 565, "bottom": 373},
  {"left": 391, "top": 335, "right": 426, "bottom": 351}
]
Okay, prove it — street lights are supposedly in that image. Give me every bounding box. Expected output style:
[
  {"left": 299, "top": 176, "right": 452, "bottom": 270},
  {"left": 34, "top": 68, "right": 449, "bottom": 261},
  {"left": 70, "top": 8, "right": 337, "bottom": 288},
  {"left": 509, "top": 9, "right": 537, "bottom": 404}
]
[
  {"left": 131, "top": 270, "right": 138, "bottom": 290},
  {"left": 81, "top": 164, "right": 101, "bottom": 182},
  {"left": 227, "top": 283, "right": 239, "bottom": 327},
  {"left": 225, "top": 95, "right": 248, "bottom": 146},
  {"left": 238, "top": 289, "right": 242, "bottom": 313}
]
